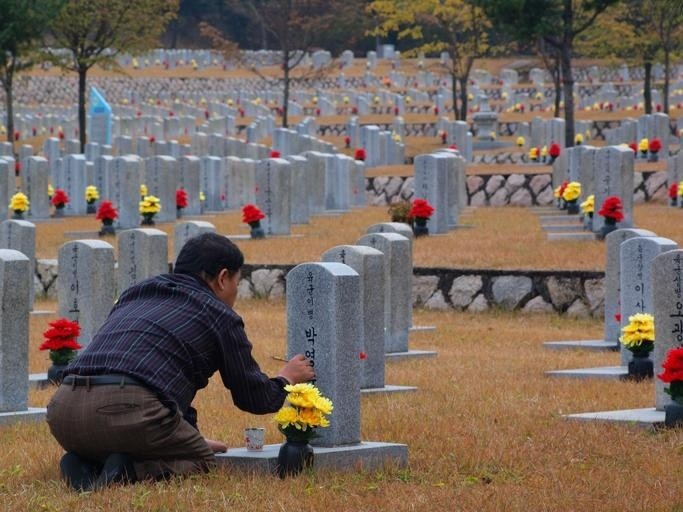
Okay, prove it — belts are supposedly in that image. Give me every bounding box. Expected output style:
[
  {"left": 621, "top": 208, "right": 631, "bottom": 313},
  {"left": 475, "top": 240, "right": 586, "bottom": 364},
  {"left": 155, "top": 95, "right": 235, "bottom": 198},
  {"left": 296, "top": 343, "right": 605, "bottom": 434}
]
[{"left": 63, "top": 374, "right": 138, "bottom": 387}]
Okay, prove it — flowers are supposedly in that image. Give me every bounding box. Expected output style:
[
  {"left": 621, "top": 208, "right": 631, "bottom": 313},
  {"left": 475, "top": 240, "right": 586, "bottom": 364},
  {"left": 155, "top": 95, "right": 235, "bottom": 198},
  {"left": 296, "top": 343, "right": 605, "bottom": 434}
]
[
  {"left": 670, "top": 183, "right": 683, "bottom": 209},
  {"left": 554, "top": 180, "right": 623, "bottom": 239},
  {"left": 37, "top": 318, "right": 82, "bottom": 364},
  {"left": 656, "top": 348, "right": 683, "bottom": 381},
  {"left": 51, "top": 184, "right": 207, "bottom": 218},
  {"left": 616, "top": 312, "right": 654, "bottom": 353},
  {"left": 7, "top": 190, "right": 31, "bottom": 210},
  {"left": 384, "top": 190, "right": 434, "bottom": 217},
  {"left": 240, "top": 202, "right": 268, "bottom": 220},
  {"left": 275, "top": 382, "right": 333, "bottom": 442}
]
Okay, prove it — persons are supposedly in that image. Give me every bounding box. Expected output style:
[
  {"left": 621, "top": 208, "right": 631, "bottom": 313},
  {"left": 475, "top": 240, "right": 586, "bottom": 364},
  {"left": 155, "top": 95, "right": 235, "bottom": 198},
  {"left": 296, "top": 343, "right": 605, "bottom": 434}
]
[{"left": 44, "top": 231, "right": 315, "bottom": 497}]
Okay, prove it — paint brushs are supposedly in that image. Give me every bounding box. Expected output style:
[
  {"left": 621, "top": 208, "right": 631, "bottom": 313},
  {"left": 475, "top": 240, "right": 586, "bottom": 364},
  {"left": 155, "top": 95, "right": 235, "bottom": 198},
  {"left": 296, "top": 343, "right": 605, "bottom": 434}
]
[{"left": 272, "top": 357, "right": 289, "bottom": 363}]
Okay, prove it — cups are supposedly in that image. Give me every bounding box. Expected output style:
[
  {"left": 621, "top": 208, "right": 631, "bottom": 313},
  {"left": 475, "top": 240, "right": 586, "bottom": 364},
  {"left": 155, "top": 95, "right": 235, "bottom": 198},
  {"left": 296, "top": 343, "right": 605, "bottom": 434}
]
[{"left": 245, "top": 428, "right": 266, "bottom": 452}]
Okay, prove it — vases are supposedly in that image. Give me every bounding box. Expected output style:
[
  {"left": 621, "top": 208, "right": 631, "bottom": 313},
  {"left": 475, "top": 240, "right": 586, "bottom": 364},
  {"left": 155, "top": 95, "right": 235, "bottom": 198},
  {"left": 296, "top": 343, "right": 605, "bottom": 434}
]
[
  {"left": 247, "top": 222, "right": 265, "bottom": 238},
  {"left": 144, "top": 214, "right": 153, "bottom": 224},
  {"left": 277, "top": 435, "right": 314, "bottom": 480},
  {"left": 102, "top": 219, "right": 113, "bottom": 226},
  {"left": 10, "top": 209, "right": 28, "bottom": 221},
  {"left": 663, "top": 382, "right": 682, "bottom": 428},
  {"left": 629, "top": 354, "right": 654, "bottom": 381},
  {"left": 392, "top": 218, "right": 429, "bottom": 237},
  {"left": 47, "top": 353, "right": 70, "bottom": 382}
]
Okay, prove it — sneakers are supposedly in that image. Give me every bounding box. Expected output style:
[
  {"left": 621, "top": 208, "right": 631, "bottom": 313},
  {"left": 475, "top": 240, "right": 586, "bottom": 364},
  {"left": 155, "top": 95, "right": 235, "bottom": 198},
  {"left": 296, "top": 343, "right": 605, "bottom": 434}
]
[
  {"left": 90, "top": 453, "right": 136, "bottom": 492},
  {"left": 60, "top": 452, "right": 90, "bottom": 493}
]
